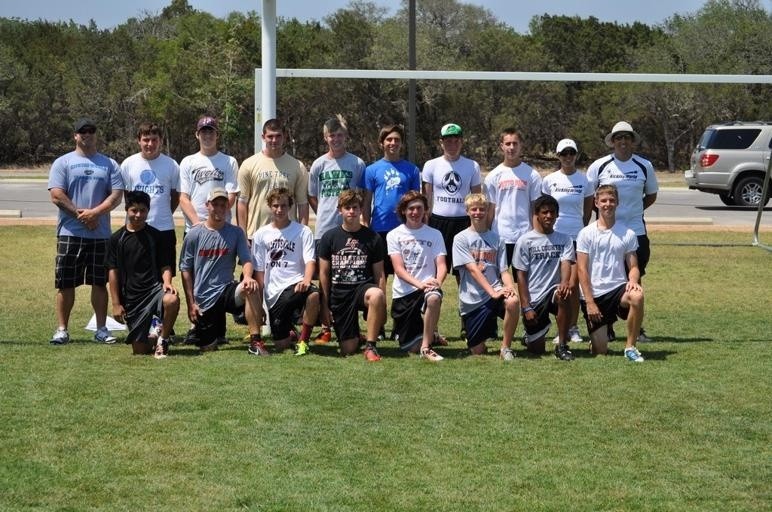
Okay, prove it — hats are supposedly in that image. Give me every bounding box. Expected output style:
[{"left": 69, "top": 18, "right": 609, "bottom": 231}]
[
  {"left": 74, "top": 118, "right": 96, "bottom": 133},
  {"left": 556, "top": 139, "right": 578, "bottom": 154},
  {"left": 208, "top": 187, "right": 229, "bottom": 202},
  {"left": 323, "top": 115, "right": 349, "bottom": 136},
  {"left": 605, "top": 121, "right": 640, "bottom": 148},
  {"left": 441, "top": 123, "right": 462, "bottom": 138},
  {"left": 197, "top": 117, "right": 219, "bottom": 134}
]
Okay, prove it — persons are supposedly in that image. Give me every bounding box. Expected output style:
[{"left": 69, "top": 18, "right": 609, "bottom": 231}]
[
  {"left": 386, "top": 190, "right": 448, "bottom": 362},
  {"left": 513, "top": 194, "right": 577, "bottom": 361},
  {"left": 251, "top": 186, "right": 320, "bottom": 357},
  {"left": 46, "top": 117, "right": 123, "bottom": 346},
  {"left": 586, "top": 121, "right": 658, "bottom": 345},
  {"left": 421, "top": 123, "right": 482, "bottom": 288},
  {"left": 177, "top": 117, "right": 240, "bottom": 346},
  {"left": 482, "top": 126, "right": 542, "bottom": 348},
  {"left": 319, "top": 190, "right": 387, "bottom": 363},
  {"left": 451, "top": 192, "right": 520, "bottom": 363},
  {"left": 575, "top": 183, "right": 645, "bottom": 364},
  {"left": 362, "top": 126, "right": 421, "bottom": 343},
  {"left": 540, "top": 139, "right": 596, "bottom": 347},
  {"left": 235, "top": 119, "right": 310, "bottom": 284},
  {"left": 178, "top": 187, "right": 272, "bottom": 357},
  {"left": 120, "top": 120, "right": 181, "bottom": 343},
  {"left": 307, "top": 114, "right": 366, "bottom": 345},
  {"left": 105, "top": 190, "right": 181, "bottom": 359}
]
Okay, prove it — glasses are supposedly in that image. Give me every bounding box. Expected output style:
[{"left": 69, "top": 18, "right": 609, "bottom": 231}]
[
  {"left": 78, "top": 128, "right": 95, "bottom": 134},
  {"left": 559, "top": 149, "right": 577, "bottom": 156},
  {"left": 614, "top": 134, "right": 631, "bottom": 140}
]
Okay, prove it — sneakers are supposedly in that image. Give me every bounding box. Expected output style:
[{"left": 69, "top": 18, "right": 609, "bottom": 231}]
[
  {"left": 624, "top": 347, "right": 644, "bottom": 362},
  {"left": 390, "top": 327, "right": 399, "bottom": 340},
  {"left": 569, "top": 326, "right": 582, "bottom": 342},
  {"left": 314, "top": 328, "right": 331, "bottom": 344},
  {"left": 500, "top": 348, "right": 513, "bottom": 360},
  {"left": 185, "top": 328, "right": 200, "bottom": 345},
  {"left": 293, "top": 341, "right": 309, "bottom": 356},
  {"left": 292, "top": 324, "right": 302, "bottom": 340},
  {"left": 554, "top": 345, "right": 575, "bottom": 361},
  {"left": 376, "top": 325, "right": 385, "bottom": 341},
  {"left": 553, "top": 333, "right": 559, "bottom": 343},
  {"left": 607, "top": 327, "right": 615, "bottom": 341},
  {"left": 420, "top": 347, "right": 444, "bottom": 360},
  {"left": 364, "top": 347, "right": 380, "bottom": 361},
  {"left": 50, "top": 329, "right": 69, "bottom": 345},
  {"left": 248, "top": 340, "right": 272, "bottom": 356},
  {"left": 152, "top": 318, "right": 176, "bottom": 343},
  {"left": 636, "top": 334, "right": 652, "bottom": 342},
  {"left": 434, "top": 331, "right": 448, "bottom": 346},
  {"left": 154, "top": 336, "right": 169, "bottom": 359},
  {"left": 94, "top": 327, "right": 117, "bottom": 344},
  {"left": 243, "top": 334, "right": 252, "bottom": 342}
]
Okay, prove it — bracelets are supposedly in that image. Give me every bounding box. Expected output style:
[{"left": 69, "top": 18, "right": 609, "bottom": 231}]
[{"left": 523, "top": 307, "right": 533, "bottom": 312}]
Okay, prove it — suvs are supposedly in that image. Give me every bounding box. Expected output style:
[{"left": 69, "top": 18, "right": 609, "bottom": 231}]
[{"left": 683, "top": 121, "right": 772, "bottom": 208}]
